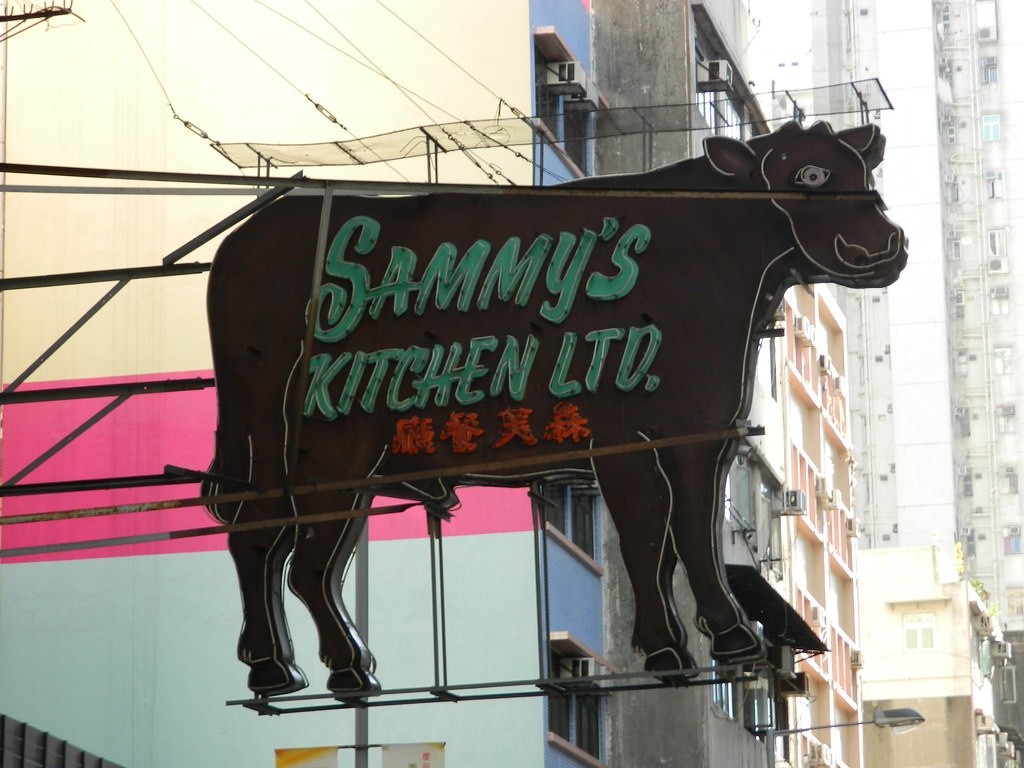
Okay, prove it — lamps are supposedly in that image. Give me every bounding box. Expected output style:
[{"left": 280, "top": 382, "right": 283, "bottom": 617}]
[{"left": 774, "top": 710, "right": 924, "bottom": 737}]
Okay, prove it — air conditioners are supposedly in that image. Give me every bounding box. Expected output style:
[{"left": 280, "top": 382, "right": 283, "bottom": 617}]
[
  {"left": 564, "top": 657, "right": 603, "bottom": 690},
  {"left": 851, "top": 650, "right": 862, "bottom": 668},
  {"left": 546, "top": 61, "right": 586, "bottom": 95},
  {"left": 698, "top": 60, "right": 732, "bottom": 90},
  {"left": 780, "top": 490, "right": 807, "bottom": 513},
  {"left": 821, "top": 355, "right": 831, "bottom": 375},
  {"left": 789, "top": 673, "right": 810, "bottom": 695},
  {"left": 994, "top": 641, "right": 1012, "bottom": 659}
]
